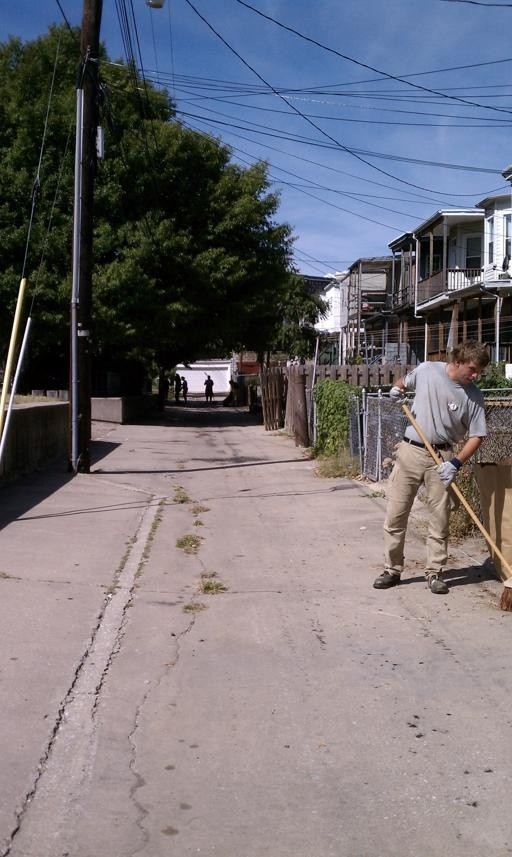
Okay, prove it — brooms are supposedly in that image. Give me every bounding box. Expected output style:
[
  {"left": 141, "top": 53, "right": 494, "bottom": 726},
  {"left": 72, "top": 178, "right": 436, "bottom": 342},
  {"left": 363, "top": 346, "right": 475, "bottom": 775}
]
[{"left": 400, "top": 401, "right": 512, "bottom": 612}]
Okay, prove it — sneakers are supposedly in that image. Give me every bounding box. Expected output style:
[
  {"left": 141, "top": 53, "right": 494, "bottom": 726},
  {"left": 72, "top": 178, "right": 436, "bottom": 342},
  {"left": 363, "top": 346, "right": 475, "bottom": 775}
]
[
  {"left": 373, "top": 572, "right": 400, "bottom": 589},
  {"left": 427, "top": 568, "right": 449, "bottom": 594}
]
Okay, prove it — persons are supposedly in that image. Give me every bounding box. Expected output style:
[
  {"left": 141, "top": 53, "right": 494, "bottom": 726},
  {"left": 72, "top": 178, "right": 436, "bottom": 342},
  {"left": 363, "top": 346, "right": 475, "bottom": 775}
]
[
  {"left": 173, "top": 373, "right": 181, "bottom": 401},
  {"left": 372, "top": 335, "right": 492, "bottom": 594},
  {"left": 204, "top": 375, "right": 213, "bottom": 402},
  {"left": 181, "top": 376, "right": 188, "bottom": 404}
]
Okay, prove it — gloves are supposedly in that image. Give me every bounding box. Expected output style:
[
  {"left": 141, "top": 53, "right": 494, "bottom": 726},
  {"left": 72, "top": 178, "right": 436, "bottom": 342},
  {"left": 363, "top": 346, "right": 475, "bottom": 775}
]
[
  {"left": 390, "top": 386, "right": 407, "bottom": 407},
  {"left": 436, "top": 457, "right": 462, "bottom": 489}
]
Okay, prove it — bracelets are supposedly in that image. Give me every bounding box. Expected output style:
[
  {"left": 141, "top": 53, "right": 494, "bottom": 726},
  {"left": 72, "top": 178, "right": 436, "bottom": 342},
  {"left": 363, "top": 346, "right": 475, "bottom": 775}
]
[{"left": 449, "top": 457, "right": 464, "bottom": 470}]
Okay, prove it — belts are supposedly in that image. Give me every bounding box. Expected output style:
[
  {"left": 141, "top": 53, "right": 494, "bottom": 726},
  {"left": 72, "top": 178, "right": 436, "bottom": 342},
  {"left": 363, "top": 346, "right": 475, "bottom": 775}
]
[{"left": 403, "top": 436, "right": 446, "bottom": 451}]
